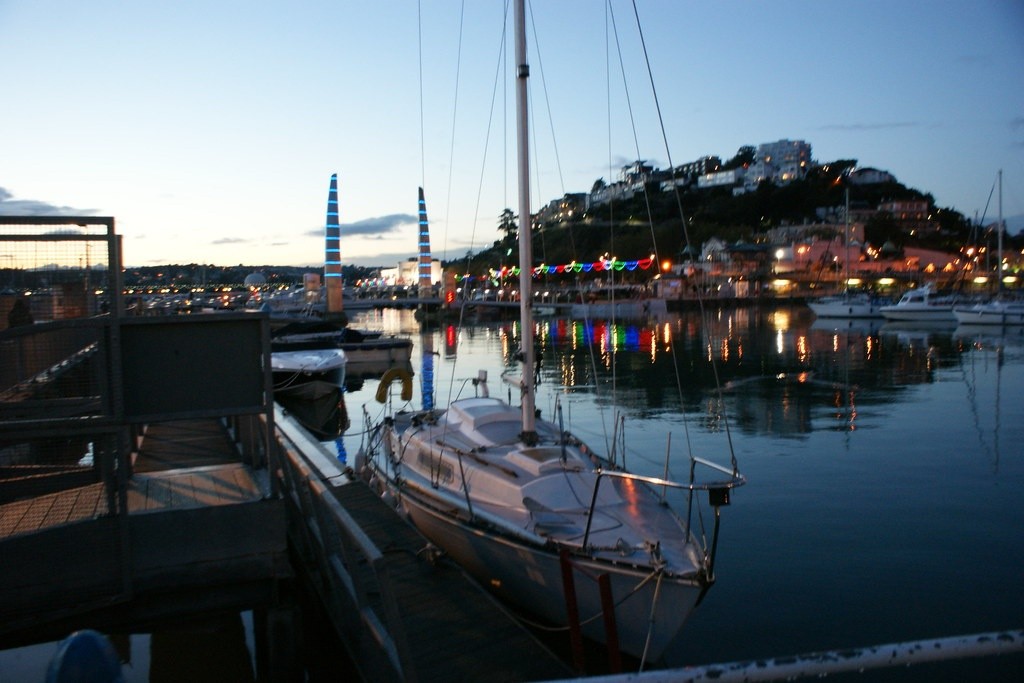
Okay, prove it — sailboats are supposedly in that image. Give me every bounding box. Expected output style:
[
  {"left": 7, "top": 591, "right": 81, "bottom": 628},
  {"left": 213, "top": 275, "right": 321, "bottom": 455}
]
[
  {"left": 349, "top": 1, "right": 748, "bottom": 679},
  {"left": 805, "top": 166, "right": 1024, "bottom": 329}
]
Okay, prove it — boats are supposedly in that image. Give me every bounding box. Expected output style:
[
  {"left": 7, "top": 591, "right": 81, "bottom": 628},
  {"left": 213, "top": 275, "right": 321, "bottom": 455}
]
[{"left": 119, "top": 275, "right": 415, "bottom": 406}]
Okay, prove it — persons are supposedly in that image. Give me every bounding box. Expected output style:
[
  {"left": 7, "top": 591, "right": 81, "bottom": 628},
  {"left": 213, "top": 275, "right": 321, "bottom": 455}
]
[{"left": 9, "top": 298, "right": 33, "bottom": 328}]
